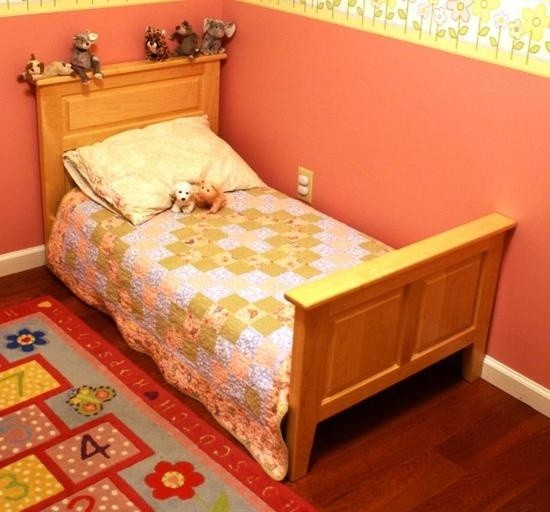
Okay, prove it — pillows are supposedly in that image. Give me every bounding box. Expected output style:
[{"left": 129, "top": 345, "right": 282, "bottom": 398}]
[{"left": 62, "top": 114, "right": 268, "bottom": 225}]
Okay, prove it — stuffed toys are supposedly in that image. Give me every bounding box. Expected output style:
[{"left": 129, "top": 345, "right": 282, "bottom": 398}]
[
  {"left": 192, "top": 180, "right": 227, "bottom": 213},
  {"left": 16, "top": 54, "right": 73, "bottom": 85},
  {"left": 73, "top": 31, "right": 104, "bottom": 86},
  {"left": 200, "top": 17, "right": 237, "bottom": 55},
  {"left": 145, "top": 26, "right": 171, "bottom": 62},
  {"left": 172, "top": 21, "right": 198, "bottom": 60},
  {"left": 170, "top": 181, "right": 194, "bottom": 213}
]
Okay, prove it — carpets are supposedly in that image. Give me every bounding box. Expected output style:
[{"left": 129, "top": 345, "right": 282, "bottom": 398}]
[{"left": 0, "top": 295, "right": 320, "bottom": 512}]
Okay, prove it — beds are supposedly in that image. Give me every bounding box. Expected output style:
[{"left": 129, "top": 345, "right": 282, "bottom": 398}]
[{"left": 34, "top": 53, "right": 519, "bottom": 483}]
